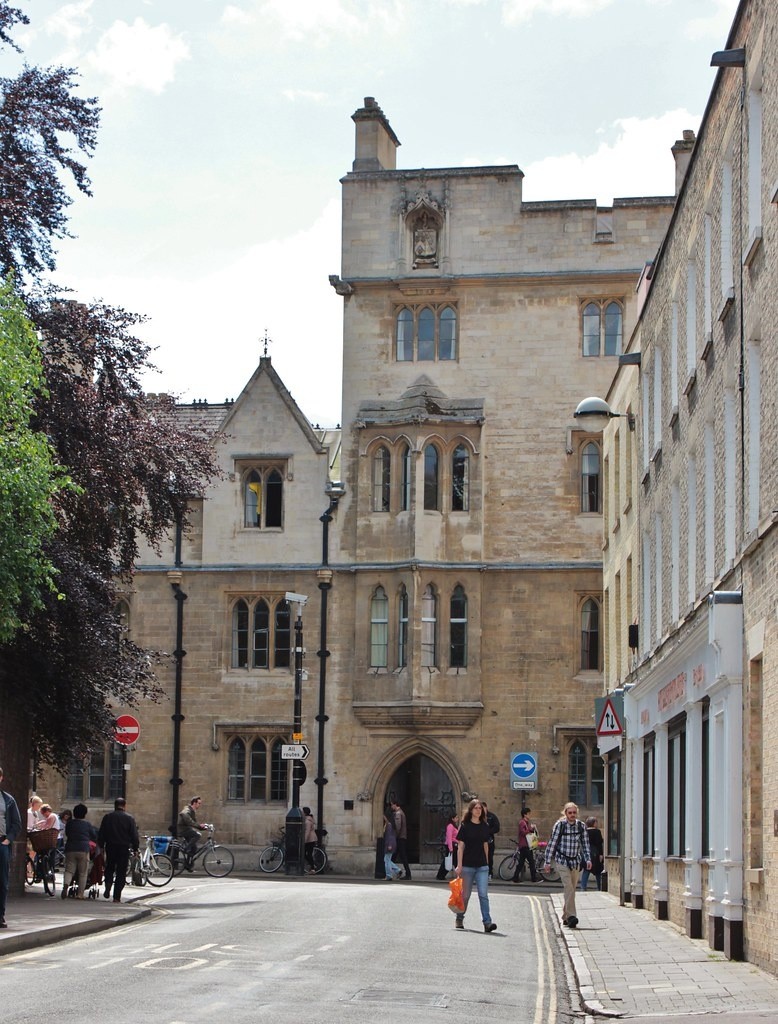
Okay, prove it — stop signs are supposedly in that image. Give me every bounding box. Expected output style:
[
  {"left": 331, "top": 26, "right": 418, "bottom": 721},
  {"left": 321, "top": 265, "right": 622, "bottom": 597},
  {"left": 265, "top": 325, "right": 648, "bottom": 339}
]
[{"left": 113, "top": 715, "right": 140, "bottom": 745}]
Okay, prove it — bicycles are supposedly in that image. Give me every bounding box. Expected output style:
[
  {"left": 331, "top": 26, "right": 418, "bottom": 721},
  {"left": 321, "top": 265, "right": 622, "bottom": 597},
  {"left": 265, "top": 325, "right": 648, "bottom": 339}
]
[
  {"left": 498, "top": 839, "right": 561, "bottom": 882},
  {"left": 26, "top": 828, "right": 174, "bottom": 897},
  {"left": 156, "top": 824, "right": 236, "bottom": 878},
  {"left": 259, "top": 826, "right": 326, "bottom": 875}
]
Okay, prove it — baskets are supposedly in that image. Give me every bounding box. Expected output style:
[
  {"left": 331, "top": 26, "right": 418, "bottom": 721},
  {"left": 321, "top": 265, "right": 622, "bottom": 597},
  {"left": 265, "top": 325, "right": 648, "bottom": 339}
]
[{"left": 28, "top": 828, "right": 60, "bottom": 852}]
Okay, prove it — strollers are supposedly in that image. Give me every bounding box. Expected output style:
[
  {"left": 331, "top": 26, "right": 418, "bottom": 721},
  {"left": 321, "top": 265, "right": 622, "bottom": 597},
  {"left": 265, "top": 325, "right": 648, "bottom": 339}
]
[{"left": 68, "top": 841, "right": 100, "bottom": 899}]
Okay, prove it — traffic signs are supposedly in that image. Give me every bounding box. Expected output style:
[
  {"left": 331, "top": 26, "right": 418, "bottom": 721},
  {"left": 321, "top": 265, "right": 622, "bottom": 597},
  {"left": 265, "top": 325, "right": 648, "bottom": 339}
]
[
  {"left": 510, "top": 751, "right": 539, "bottom": 791},
  {"left": 281, "top": 744, "right": 309, "bottom": 760}
]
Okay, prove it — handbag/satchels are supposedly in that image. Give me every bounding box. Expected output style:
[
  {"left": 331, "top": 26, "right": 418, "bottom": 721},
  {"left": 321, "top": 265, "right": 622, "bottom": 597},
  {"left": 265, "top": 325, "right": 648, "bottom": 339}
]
[
  {"left": 445, "top": 852, "right": 453, "bottom": 872},
  {"left": 526, "top": 833, "right": 538, "bottom": 850},
  {"left": 447, "top": 875, "right": 465, "bottom": 913}
]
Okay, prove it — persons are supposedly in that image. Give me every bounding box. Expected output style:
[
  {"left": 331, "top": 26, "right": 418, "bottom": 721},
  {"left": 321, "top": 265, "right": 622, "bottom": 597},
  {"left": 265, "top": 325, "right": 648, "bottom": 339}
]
[
  {"left": 0, "top": 768, "right": 22, "bottom": 928},
  {"left": 512, "top": 807, "right": 544, "bottom": 883},
  {"left": 482, "top": 802, "right": 500, "bottom": 879},
  {"left": 390, "top": 800, "right": 413, "bottom": 880},
  {"left": 582, "top": 816, "right": 604, "bottom": 891},
  {"left": 382, "top": 812, "right": 402, "bottom": 880},
  {"left": 455, "top": 799, "right": 497, "bottom": 933},
  {"left": 544, "top": 802, "right": 592, "bottom": 928},
  {"left": 178, "top": 796, "right": 205, "bottom": 872},
  {"left": 98, "top": 798, "right": 140, "bottom": 902},
  {"left": 303, "top": 807, "right": 317, "bottom": 874},
  {"left": 27, "top": 796, "right": 98, "bottom": 900},
  {"left": 436, "top": 813, "right": 459, "bottom": 881}
]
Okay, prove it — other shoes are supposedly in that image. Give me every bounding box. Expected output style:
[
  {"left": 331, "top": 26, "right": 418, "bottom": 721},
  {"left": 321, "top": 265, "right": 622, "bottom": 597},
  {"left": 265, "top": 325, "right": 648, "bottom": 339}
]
[
  {"left": 60, "top": 887, "right": 68, "bottom": 900},
  {"left": 305, "top": 870, "right": 316, "bottom": 875},
  {"left": 103, "top": 888, "right": 110, "bottom": 898},
  {"left": 0, "top": 914, "right": 7, "bottom": 928},
  {"left": 488, "top": 875, "right": 493, "bottom": 882},
  {"left": 399, "top": 875, "right": 412, "bottom": 880},
  {"left": 456, "top": 919, "right": 464, "bottom": 929},
  {"left": 510, "top": 877, "right": 520, "bottom": 883},
  {"left": 436, "top": 876, "right": 447, "bottom": 880},
  {"left": 113, "top": 898, "right": 121, "bottom": 902},
  {"left": 384, "top": 878, "right": 392, "bottom": 881},
  {"left": 396, "top": 871, "right": 402, "bottom": 879},
  {"left": 532, "top": 879, "right": 543, "bottom": 883},
  {"left": 563, "top": 916, "right": 578, "bottom": 928},
  {"left": 484, "top": 923, "right": 497, "bottom": 932}
]
